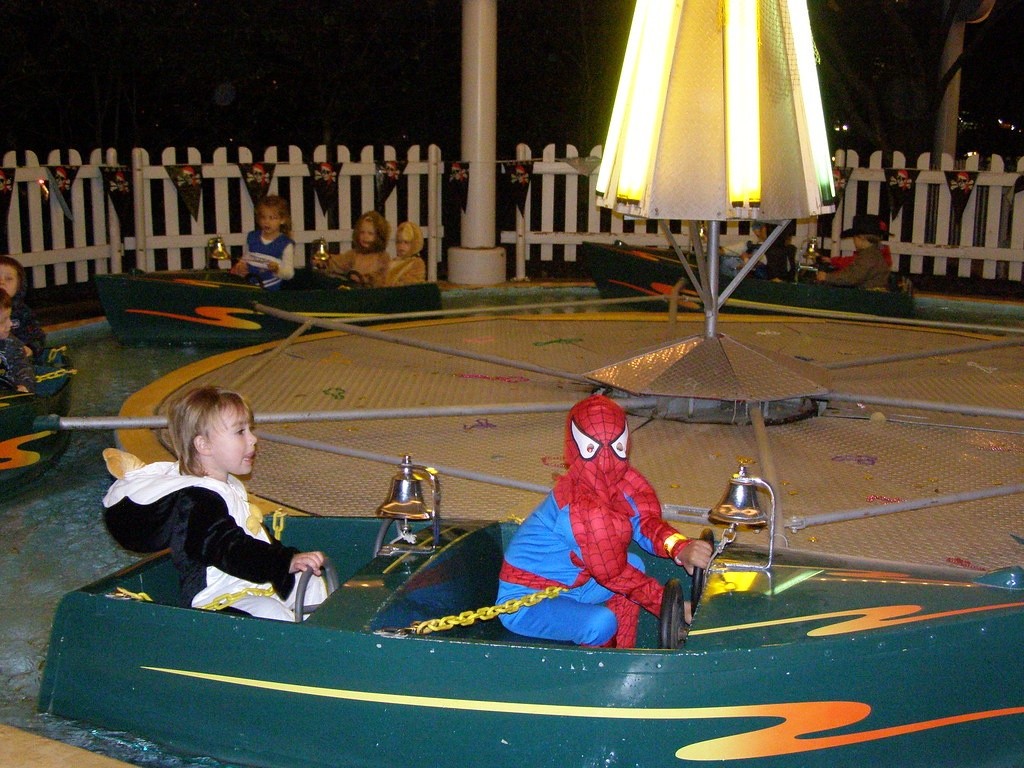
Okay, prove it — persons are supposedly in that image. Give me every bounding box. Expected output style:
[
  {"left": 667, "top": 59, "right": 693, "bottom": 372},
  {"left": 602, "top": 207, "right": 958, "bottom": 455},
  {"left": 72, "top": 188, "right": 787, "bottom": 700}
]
[
  {"left": 315, "top": 211, "right": 425, "bottom": 287},
  {"left": 719, "top": 212, "right": 894, "bottom": 292},
  {"left": 230, "top": 192, "right": 295, "bottom": 290},
  {"left": 0, "top": 257, "right": 47, "bottom": 393},
  {"left": 496, "top": 395, "right": 713, "bottom": 651},
  {"left": 101, "top": 389, "right": 327, "bottom": 623}
]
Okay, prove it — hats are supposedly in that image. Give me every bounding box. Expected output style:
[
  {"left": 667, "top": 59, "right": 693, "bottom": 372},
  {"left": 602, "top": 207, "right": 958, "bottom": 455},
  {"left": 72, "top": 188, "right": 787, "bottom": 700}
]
[{"left": 839, "top": 214, "right": 895, "bottom": 239}]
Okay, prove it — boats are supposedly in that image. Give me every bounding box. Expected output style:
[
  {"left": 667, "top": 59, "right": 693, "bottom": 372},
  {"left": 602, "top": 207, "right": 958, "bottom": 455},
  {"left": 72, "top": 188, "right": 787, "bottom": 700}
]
[
  {"left": 1, "top": 345, "right": 74, "bottom": 500},
  {"left": 33, "top": 513, "right": 1024, "bottom": 768},
  {"left": 580, "top": 239, "right": 914, "bottom": 322},
  {"left": 90, "top": 261, "right": 445, "bottom": 349}
]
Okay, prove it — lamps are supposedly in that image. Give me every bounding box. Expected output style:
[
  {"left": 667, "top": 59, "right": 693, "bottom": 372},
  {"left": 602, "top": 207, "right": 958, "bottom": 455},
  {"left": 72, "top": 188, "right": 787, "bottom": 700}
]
[{"left": 594, "top": 0, "right": 836, "bottom": 218}]
